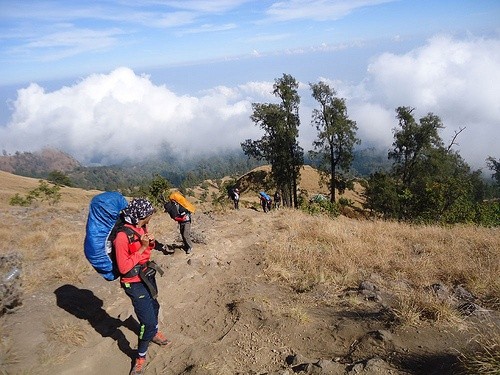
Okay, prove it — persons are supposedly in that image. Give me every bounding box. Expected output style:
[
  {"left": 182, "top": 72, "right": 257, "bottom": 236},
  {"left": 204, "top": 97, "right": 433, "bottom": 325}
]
[
  {"left": 113, "top": 196, "right": 172, "bottom": 375},
  {"left": 258, "top": 188, "right": 281, "bottom": 213},
  {"left": 168, "top": 192, "right": 194, "bottom": 254},
  {"left": 226, "top": 186, "right": 241, "bottom": 208}
]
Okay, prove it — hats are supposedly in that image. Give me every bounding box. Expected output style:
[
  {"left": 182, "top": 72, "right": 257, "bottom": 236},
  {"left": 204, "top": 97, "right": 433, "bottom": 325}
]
[
  {"left": 164, "top": 202, "right": 175, "bottom": 213},
  {"left": 235, "top": 189, "right": 239, "bottom": 192}
]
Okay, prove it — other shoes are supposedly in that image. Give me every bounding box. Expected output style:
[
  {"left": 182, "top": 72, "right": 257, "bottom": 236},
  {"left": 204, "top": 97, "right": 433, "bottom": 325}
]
[{"left": 187, "top": 247, "right": 193, "bottom": 253}]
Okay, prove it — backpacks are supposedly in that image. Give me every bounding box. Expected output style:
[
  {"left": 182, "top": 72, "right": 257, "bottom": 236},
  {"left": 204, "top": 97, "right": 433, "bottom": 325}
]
[
  {"left": 259, "top": 192, "right": 270, "bottom": 200},
  {"left": 169, "top": 192, "right": 195, "bottom": 214},
  {"left": 232, "top": 192, "right": 239, "bottom": 200},
  {"left": 84, "top": 192, "right": 129, "bottom": 281}
]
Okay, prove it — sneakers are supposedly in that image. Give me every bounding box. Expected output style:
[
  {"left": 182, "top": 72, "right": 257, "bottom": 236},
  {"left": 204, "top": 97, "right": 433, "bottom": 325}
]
[
  {"left": 130, "top": 355, "right": 147, "bottom": 375},
  {"left": 152, "top": 331, "right": 172, "bottom": 348}
]
